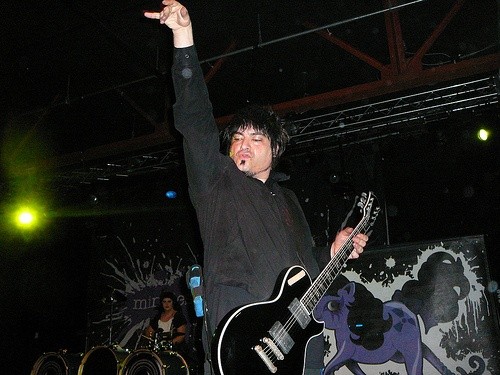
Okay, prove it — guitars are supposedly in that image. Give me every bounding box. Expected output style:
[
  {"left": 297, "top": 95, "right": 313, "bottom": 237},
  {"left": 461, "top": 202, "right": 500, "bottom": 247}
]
[{"left": 210, "top": 191, "right": 381, "bottom": 375}]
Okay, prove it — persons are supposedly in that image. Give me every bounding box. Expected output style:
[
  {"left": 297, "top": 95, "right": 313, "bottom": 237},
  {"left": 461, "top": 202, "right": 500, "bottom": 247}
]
[
  {"left": 144, "top": 0, "right": 369, "bottom": 375},
  {"left": 147, "top": 292, "right": 186, "bottom": 345}
]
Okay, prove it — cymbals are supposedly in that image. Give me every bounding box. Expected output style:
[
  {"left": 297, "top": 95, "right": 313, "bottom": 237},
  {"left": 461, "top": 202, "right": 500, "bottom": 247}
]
[{"left": 159, "top": 332, "right": 186, "bottom": 336}]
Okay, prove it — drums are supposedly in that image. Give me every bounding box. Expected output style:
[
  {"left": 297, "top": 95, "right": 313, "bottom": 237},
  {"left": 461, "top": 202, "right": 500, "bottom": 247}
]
[
  {"left": 78, "top": 345, "right": 129, "bottom": 375},
  {"left": 30, "top": 351, "right": 75, "bottom": 375},
  {"left": 118, "top": 349, "right": 190, "bottom": 375}
]
